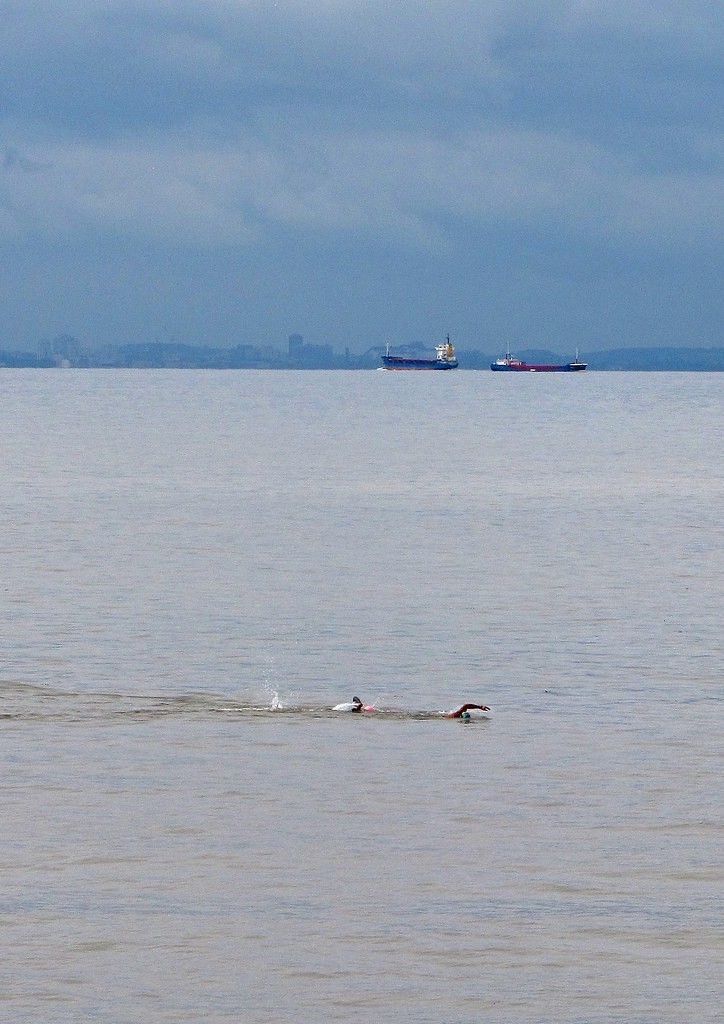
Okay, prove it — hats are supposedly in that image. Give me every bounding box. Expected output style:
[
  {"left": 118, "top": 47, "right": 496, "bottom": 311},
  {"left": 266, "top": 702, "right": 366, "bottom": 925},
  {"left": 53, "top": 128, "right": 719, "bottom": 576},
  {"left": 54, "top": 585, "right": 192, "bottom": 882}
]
[
  {"left": 364, "top": 706, "right": 374, "bottom": 712},
  {"left": 461, "top": 712, "right": 471, "bottom": 719}
]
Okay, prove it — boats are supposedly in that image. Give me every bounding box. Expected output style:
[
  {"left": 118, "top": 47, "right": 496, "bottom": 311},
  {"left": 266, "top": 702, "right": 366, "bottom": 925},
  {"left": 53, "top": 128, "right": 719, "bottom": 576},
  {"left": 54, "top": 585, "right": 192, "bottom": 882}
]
[
  {"left": 490, "top": 341, "right": 587, "bottom": 371},
  {"left": 380, "top": 333, "right": 459, "bottom": 370}
]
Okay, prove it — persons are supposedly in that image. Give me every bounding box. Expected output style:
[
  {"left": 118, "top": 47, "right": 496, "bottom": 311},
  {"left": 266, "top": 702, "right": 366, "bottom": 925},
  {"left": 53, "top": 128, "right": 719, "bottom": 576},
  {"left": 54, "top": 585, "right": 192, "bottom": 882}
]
[
  {"left": 351, "top": 696, "right": 374, "bottom": 713},
  {"left": 447, "top": 704, "right": 491, "bottom": 719}
]
